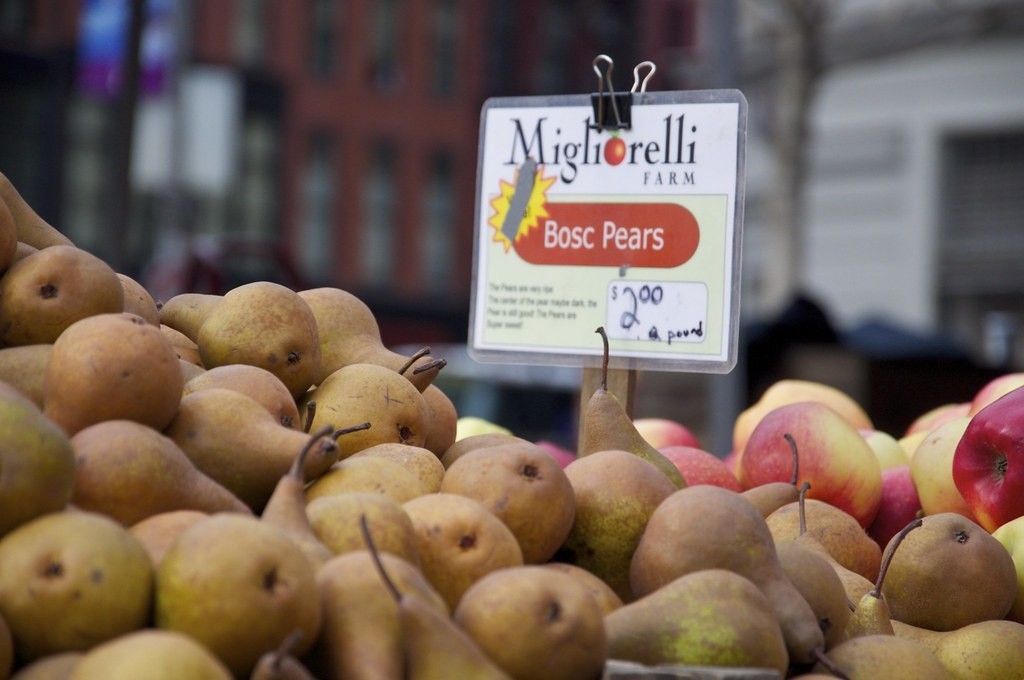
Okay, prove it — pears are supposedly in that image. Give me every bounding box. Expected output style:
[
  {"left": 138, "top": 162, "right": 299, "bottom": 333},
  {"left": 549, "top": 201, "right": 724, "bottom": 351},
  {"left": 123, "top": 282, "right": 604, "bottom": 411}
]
[
  {"left": 610, "top": 432, "right": 1024, "bottom": 680},
  {"left": 0, "top": 171, "right": 688, "bottom": 680}
]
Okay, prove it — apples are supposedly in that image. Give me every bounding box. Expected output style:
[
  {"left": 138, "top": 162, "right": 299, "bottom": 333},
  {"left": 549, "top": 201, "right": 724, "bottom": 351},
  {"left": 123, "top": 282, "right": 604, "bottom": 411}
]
[{"left": 541, "top": 368, "right": 1023, "bottom": 610}]
